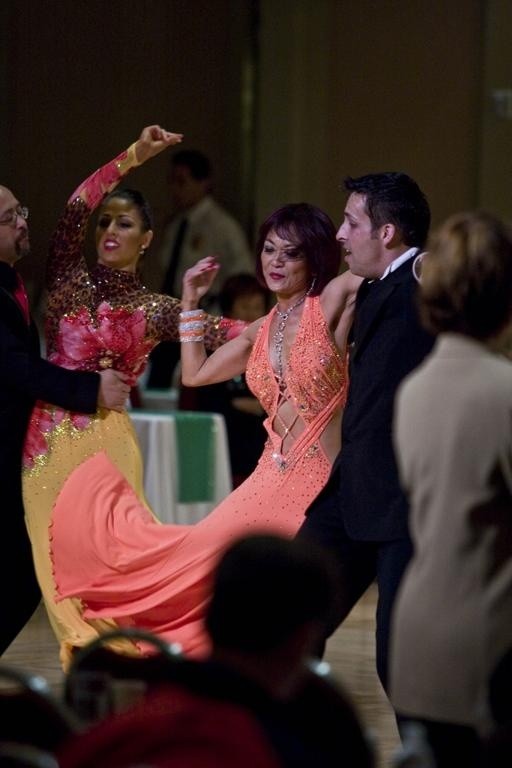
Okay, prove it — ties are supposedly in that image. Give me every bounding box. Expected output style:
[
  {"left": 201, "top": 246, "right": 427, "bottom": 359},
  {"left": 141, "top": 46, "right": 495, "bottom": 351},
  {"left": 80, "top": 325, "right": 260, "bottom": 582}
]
[{"left": 161, "top": 217, "right": 189, "bottom": 294}]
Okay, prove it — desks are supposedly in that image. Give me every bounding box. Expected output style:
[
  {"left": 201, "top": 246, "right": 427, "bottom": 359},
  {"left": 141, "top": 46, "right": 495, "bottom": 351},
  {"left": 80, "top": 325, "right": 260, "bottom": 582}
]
[{"left": 130, "top": 389, "right": 230, "bottom": 524}]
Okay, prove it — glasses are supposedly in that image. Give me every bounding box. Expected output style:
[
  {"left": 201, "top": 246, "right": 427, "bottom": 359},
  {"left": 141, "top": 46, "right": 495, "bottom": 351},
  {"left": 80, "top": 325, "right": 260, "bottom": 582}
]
[{"left": 0, "top": 206, "right": 30, "bottom": 227}]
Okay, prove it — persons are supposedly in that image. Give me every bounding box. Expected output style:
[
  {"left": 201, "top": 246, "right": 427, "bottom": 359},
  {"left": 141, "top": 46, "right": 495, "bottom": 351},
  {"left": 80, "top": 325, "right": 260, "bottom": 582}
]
[
  {"left": 0, "top": 185, "right": 130, "bottom": 657},
  {"left": 14, "top": 118, "right": 258, "bottom": 681},
  {"left": 46, "top": 171, "right": 441, "bottom": 768},
  {"left": 2, "top": 685, "right": 70, "bottom": 768},
  {"left": 392, "top": 214, "right": 512, "bottom": 768},
  {"left": 152, "top": 146, "right": 256, "bottom": 301}
]
